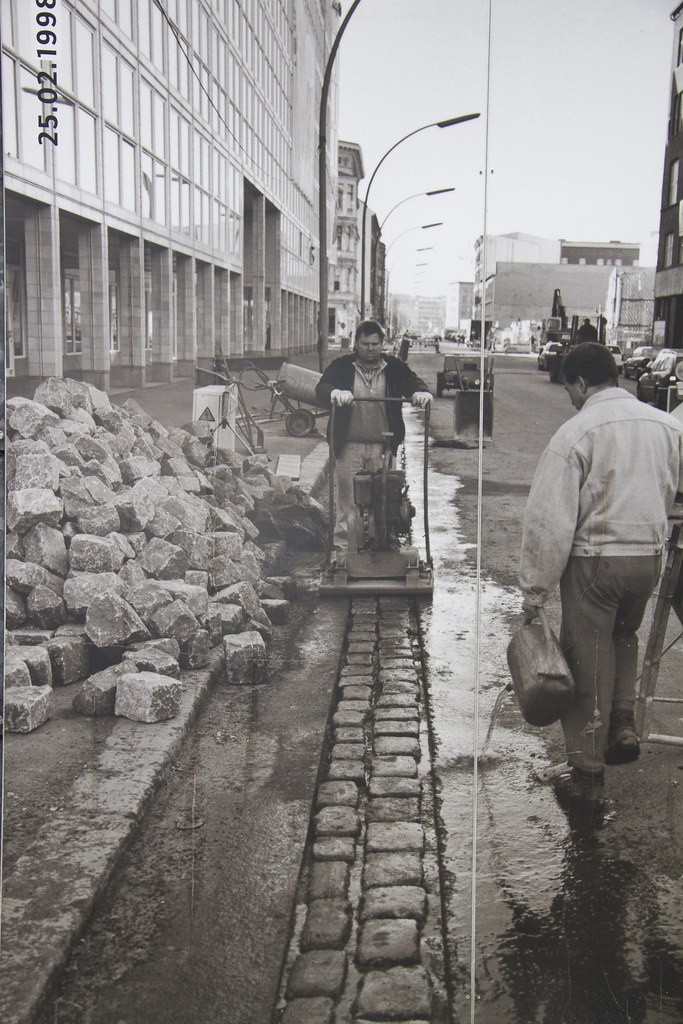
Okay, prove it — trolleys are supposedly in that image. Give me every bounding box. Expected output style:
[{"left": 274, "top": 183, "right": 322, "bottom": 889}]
[{"left": 452, "top": 356, "right": 496, "bottom": 444}]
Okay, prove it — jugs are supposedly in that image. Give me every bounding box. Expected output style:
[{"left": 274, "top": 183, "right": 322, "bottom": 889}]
[{"left": 505, "top": 607, "right": 575, "bottom": 725}]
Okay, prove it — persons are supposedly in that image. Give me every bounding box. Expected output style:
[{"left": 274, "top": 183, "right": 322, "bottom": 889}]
[
  {"left": 401, "top": 329, "right": 410, "bottom": 362},
  {"left": 514, "top": 342, "right": 683, "bottom": 811},
  {"left": 315, "top": 320, "right": 434, "bottom": 521},
  {"left": 578, "top": 318, "right": 598, "bottom": 342},
  {"left": 665, "top": 400, "right": 683, "bottom": 625}
]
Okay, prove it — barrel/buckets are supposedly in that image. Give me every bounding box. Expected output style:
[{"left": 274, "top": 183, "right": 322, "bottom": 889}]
[{"left": 276, "top": 362, "right": 330, "bottom": 410}]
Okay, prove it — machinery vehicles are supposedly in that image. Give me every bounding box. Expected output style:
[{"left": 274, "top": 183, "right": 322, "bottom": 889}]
[{"left": 538, "top": 287, "right": 608, "bottom": 382}]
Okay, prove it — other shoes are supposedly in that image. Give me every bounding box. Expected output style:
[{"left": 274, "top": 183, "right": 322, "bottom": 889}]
[
  {"left": 609, "top": 729, "right": 639, "bottom": 763},
  {"left": 555, "top": 774, "right": 604, "bottom": 812}
]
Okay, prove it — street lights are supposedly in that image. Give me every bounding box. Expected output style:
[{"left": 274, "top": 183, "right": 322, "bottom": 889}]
[
  {"left": 360, "top": 112, "right": 479, "bottom": 324},
  {"left": 370, "top": 186, "right": 456, "bottom": 340}
]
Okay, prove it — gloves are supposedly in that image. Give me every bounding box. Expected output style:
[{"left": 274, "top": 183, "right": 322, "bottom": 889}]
[
  {"left": 330, "top": 389, "right": 355, "bottom": 408},
  {"left": 411, "top": 391, "right": 433, "bottom": 409}
]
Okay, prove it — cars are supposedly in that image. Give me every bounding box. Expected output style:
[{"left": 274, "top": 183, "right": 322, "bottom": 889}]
[
  {"left": 537, "top": 341, "right": 563, "bottom": 369},
  {"left": 604, "top": 345, "right": 624, "bottom": 374},
  {"left": 635, "top": 347, "right": 683, "bottom": 410},
  {"left": 622, "top": 346, "right": 662, "bottom": 379}
]
